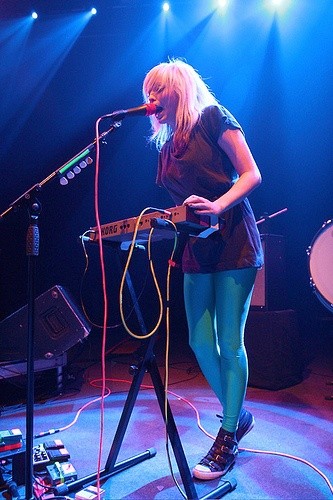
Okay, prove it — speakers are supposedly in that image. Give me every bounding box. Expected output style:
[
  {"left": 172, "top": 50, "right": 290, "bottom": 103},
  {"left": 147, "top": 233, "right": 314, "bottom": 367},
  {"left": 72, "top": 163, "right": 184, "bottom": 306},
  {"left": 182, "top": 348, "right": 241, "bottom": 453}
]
[
  {"left": 1, "top": 285, "right": 93, "bottom": 365},
  {"left": 250, "top": 239, "right": 268, "bottom": 308}
]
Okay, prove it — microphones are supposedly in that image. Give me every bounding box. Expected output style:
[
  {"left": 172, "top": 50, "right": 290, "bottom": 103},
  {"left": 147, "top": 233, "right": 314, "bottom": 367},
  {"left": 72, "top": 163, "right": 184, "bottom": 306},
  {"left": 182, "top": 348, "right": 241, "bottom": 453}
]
[{"left": 109, "top": 103, "right": 157, "bottom": 118}]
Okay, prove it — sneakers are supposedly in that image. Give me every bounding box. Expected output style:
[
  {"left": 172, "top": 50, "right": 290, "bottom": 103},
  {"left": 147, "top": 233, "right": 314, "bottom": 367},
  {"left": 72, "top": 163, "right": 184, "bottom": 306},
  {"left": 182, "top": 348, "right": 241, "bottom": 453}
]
[
  {"left": 217, "top": 409, "right": 255, "bottom": 442},
  {"left": 192, "top": 427, "right": 239, "bottom": 480}
]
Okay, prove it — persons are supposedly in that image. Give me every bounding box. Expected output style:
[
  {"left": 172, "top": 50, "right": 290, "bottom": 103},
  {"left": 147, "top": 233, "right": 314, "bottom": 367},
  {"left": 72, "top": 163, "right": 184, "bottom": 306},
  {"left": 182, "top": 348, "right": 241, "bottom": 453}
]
[{"left": 143, "top": 59, "right": 266, "bottom": 483}]
